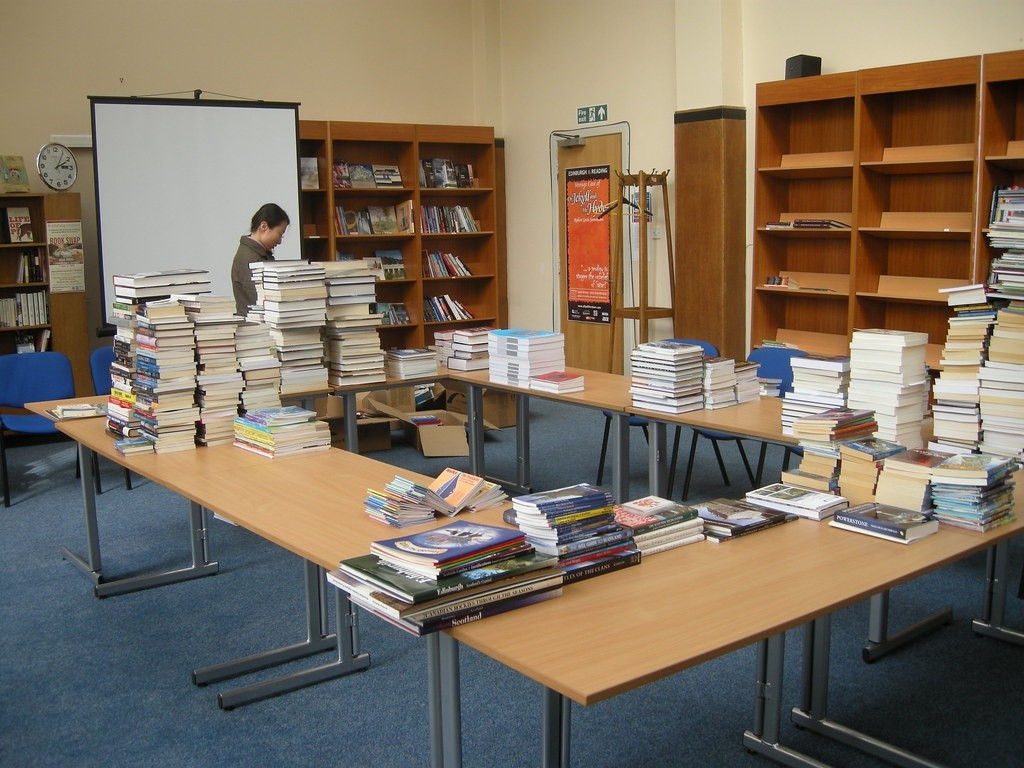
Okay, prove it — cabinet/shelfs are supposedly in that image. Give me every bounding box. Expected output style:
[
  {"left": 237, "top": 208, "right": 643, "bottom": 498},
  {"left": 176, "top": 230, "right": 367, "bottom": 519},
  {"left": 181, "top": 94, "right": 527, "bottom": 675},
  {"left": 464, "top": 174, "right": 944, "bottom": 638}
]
[
  {"left": 299, "top": 118, "right": 509, "bottom": 351},
  {"left": 0, "top": 192, "right": 97, "bottom": 438},
  {"left": 744, "top": 49, "right": 1024, "bottom": 371}
]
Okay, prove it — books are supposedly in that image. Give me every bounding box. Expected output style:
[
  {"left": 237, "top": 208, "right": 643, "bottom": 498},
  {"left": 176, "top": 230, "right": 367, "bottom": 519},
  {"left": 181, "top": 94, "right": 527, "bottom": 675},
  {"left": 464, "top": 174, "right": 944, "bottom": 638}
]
[
  {"left": 104, "top": 251, "right": 299, "bottom": 461},
  {"left": 300, "top": 156, "right": 1024, "bottom": 639},
  {"left": 1, "top": 154, "right": 54, "bottom": 360},
  {"left": 299, "top": 156, "right": 482, "bottom": 236}
]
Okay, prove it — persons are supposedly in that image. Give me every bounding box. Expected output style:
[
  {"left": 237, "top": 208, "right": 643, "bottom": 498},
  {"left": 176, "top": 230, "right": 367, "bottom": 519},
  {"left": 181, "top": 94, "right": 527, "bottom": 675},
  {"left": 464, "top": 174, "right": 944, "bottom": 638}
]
[{"left": 230, "top": 203, "right": 291, "bottom": 317}]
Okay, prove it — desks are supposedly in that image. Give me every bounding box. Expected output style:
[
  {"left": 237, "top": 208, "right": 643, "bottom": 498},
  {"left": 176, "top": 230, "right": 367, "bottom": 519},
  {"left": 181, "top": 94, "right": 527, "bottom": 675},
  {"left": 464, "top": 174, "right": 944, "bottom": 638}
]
[{"left": 23, "top": 362, "right": 1024, "bottom": 766}]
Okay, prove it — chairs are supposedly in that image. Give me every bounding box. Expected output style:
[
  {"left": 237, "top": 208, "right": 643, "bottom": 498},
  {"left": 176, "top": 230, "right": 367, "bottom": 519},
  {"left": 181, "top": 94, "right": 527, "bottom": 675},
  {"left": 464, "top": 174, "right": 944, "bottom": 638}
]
[
  {"left": 89, "top": 346, "right": 132, "bottom": 490},
  {"left": 595, "top": 338, "right": 731, "bottom": 501},
  {"left": 681, "top": 347, "right": 810, "bottom": 502},
  {"left": 1, "top": 351, "right": 103, "bottom": 507}
]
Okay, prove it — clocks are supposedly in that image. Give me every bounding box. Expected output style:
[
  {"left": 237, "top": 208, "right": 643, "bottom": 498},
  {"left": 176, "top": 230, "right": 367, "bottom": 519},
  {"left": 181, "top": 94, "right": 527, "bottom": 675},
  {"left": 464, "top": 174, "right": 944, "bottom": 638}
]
[{"left": 36, "top": 142, "right": 78, "bottom": 191}]
